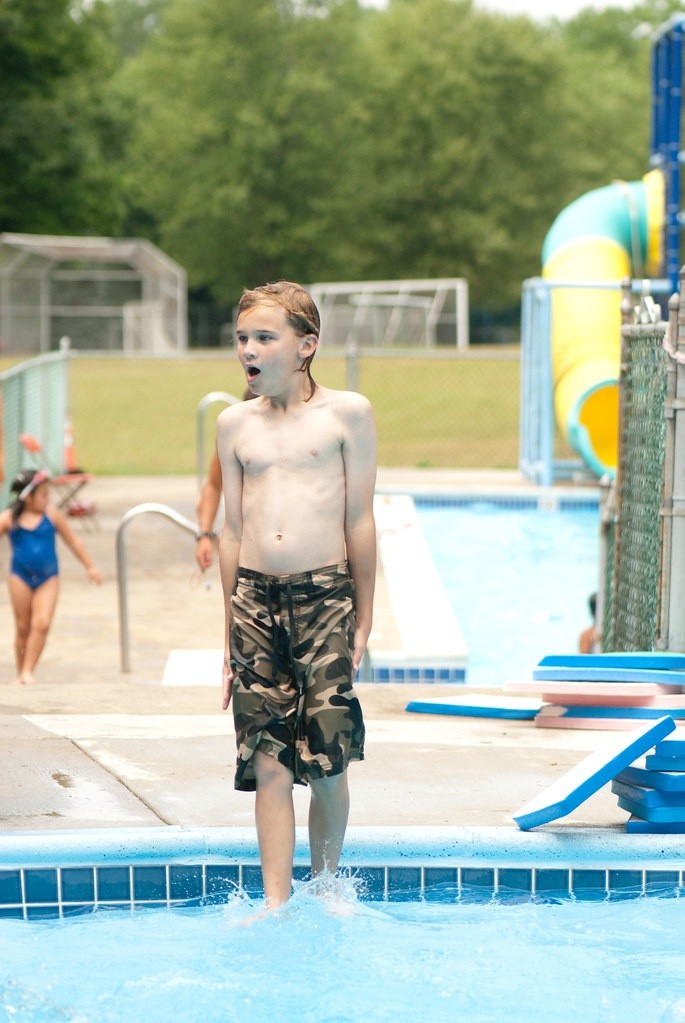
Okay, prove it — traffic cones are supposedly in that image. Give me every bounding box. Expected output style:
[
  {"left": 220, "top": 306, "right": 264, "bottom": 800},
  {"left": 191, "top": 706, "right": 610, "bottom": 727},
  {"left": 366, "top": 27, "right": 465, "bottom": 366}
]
[{"left": 65, "top": 422, "right": 83, "bottom": 474}]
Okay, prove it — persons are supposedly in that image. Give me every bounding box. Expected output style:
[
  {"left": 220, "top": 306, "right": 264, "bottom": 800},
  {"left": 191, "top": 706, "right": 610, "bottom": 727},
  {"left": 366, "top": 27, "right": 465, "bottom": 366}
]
[
  {"left": 217, "top": 279, "right": 376, "bottom": 910},
  {"left": 578, "top": 593, "right": 596, "bottom": 654},
  {"left": 0, "top": 469, "right": 103, "bottom": 683},
  {"left": 197, "top": 387, "right": 260, "bottom": 573}
]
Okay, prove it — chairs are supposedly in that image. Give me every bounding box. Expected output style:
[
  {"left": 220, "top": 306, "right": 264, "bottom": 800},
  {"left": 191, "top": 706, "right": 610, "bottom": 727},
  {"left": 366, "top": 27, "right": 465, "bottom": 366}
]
[{"left": 18, "top": 433, "right": 101, "bottom": 535}]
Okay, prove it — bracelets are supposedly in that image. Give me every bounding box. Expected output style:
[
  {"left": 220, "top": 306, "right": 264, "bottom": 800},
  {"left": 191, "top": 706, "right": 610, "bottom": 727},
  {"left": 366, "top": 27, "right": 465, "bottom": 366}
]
[{"left": 197, "top": 532, "right": 215, "bottom": 540}]
